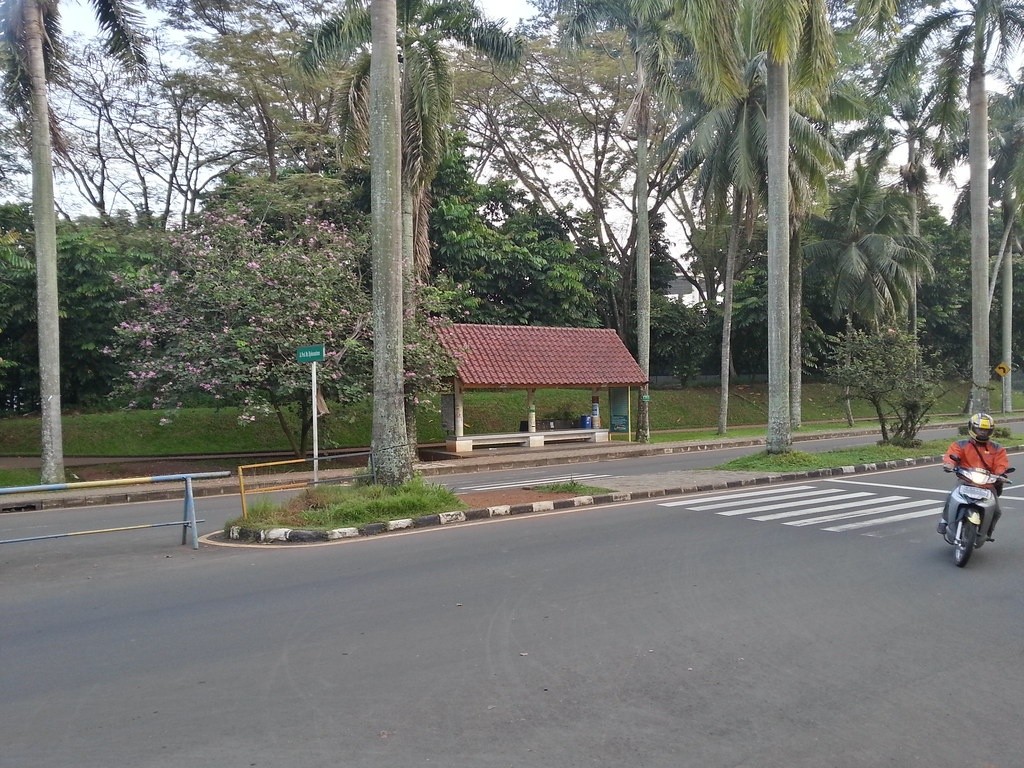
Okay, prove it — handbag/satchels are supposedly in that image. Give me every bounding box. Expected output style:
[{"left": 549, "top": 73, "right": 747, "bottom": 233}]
[{"left": 995, "top": 481, "right": 1002, "bottom": 496}]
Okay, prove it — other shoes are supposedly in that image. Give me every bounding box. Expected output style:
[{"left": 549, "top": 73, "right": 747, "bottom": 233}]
[
  {"left": 937, "top": 523, "right": 948, "bottom": 534},
  {"left": 986, "top": 537, "right": 995, "bottom": 541}
]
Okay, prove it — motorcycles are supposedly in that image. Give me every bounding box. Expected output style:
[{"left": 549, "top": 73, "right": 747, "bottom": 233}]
[{"left": 937, "top": 454, "right": 1016, "bottom": 568}]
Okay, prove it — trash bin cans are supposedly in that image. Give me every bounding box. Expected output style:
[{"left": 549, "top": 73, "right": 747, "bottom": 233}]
[
  {"left": 520, "top": 421, "right": 528, "bottom": 431},
  {"left": 581, "top": 416, "right": 592, "bottom": 429}
]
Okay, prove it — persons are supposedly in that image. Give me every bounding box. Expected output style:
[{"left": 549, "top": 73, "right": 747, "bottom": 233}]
[{"left": 937, "top": 412, "right": 1009, "bottom": 541}]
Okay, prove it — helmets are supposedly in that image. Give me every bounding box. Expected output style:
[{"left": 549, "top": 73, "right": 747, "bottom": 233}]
[{"left": 968, "top": 413, "right": 995, "bottom": 442}]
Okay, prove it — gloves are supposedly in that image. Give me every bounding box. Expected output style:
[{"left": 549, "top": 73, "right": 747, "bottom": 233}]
[{"left": 943, "top": 462, "right": 955, "bottom": 473}]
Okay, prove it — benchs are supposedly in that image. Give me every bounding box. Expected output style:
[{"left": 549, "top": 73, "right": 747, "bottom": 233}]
[{"left": 446, "top": 428, "right": 610, "bottom": 452}]
